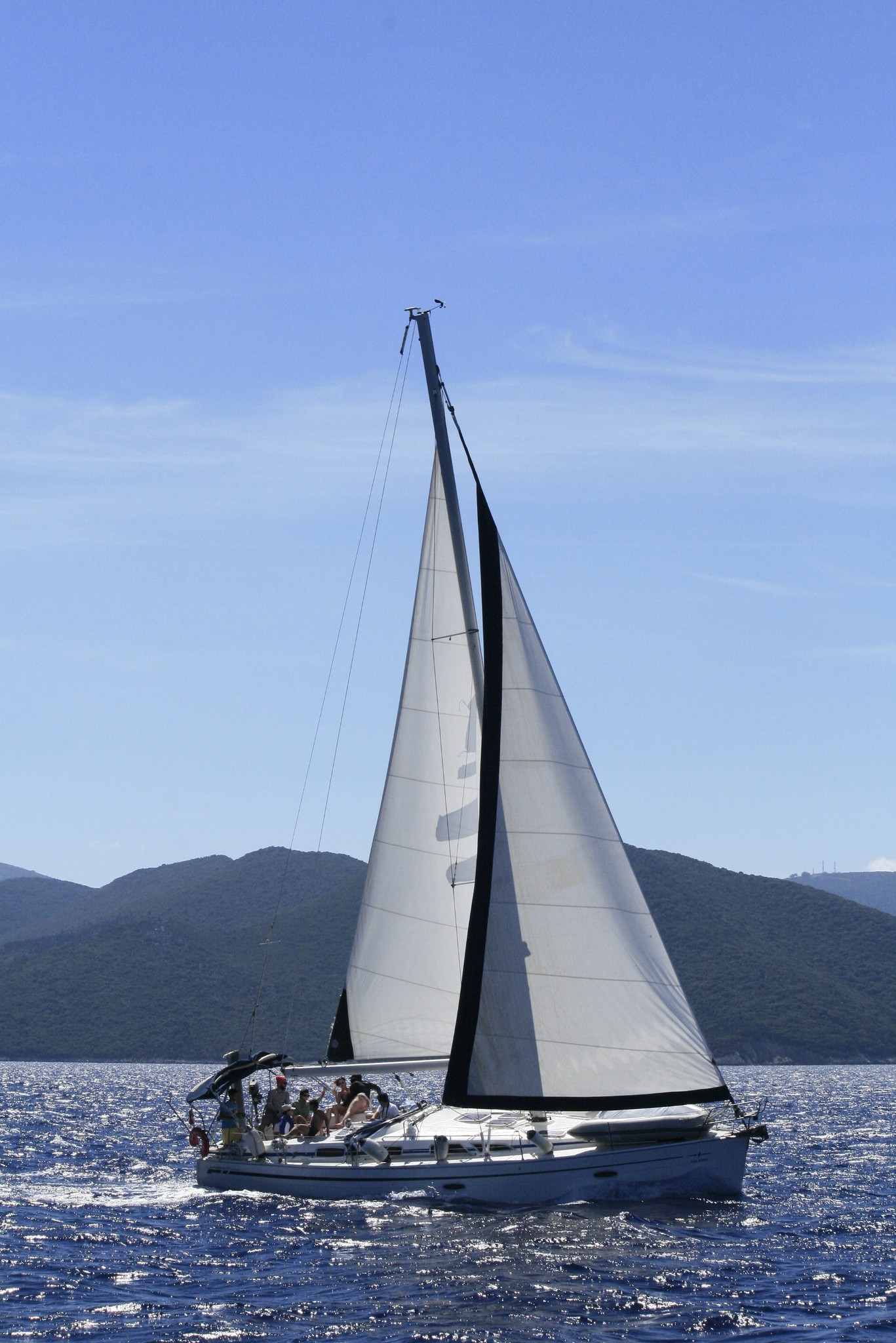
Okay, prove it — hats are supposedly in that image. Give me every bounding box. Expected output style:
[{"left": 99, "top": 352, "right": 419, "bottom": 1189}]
[
  {"left": 309, "top": 1100, "right": 319, "bottom": 1108},
  {"left": 275, "top": 1075, "right": 286, "bottom": 1084},
  {"left": 281, "top": 1105, "right": 296, "bottom": 1113},
  {"left": 378, "top": 1093, "right": 389, "bottom": 1101},
  {"left": 348, "top": 1074, "right": 362, "bottom": 1080}
]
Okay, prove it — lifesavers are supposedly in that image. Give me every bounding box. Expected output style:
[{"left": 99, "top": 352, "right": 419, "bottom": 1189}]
[{"left": 189, "top": 1127, "right": 210, "bottom": 1157}]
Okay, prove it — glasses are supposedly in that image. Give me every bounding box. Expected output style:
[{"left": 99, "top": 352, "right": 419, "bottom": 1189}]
[
  {"left": 339, "top": 1082, "right": 344, "bottom": 1085},
  {"left": 283, "top": 1081, "right": 287, "bottom": 1085},
  {"left": 304, "top": 1094, "right": 310, "bottom": 1097}
]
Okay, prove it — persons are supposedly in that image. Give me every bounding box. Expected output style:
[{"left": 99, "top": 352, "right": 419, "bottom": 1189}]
[
  {"left": 260, "top": 1075, "right": 399, "bottom": 1140},
  {"left": 218, "top": 1088, "right": 240, "bottom": 1146}
]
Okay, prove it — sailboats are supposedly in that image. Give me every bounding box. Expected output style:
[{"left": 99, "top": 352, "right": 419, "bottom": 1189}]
[{"left": 179, "top": 297, "right": 771, "bottom": 1208}]
